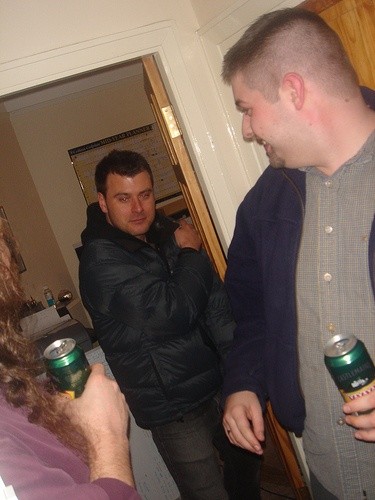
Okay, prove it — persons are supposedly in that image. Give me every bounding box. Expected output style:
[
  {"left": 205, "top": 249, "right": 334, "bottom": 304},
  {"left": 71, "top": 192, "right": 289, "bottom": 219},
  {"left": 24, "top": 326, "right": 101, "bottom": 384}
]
[
  {"left": 221, "top": 9, "right": 375, "bottom": 500},
  {"left": 0, "top": 218, "right": 139, "bottom": 500},
  {"left": 76, "top": 151, "right": 265, "bottom": 500}
]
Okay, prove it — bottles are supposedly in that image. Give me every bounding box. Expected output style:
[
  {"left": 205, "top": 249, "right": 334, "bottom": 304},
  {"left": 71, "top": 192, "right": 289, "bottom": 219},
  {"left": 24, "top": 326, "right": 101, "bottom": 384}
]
[{"left": 43, "top": 286, "right": 55, "bottom": 307}]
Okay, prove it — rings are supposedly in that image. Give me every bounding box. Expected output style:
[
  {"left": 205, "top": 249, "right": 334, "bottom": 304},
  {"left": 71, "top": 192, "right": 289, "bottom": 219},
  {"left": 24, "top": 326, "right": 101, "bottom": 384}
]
[{"left": 227, "top": 430, "right": 231, "bottom": 434}]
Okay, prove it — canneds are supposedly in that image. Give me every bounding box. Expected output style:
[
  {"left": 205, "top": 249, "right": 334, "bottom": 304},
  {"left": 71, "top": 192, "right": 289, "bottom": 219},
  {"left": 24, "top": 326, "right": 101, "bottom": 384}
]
[
  {"left": 43, "top": 337, "right": 90, "bottom": 399},
  {"left": 323, "top": 333, "right": 375, "bottom": 415}
]
